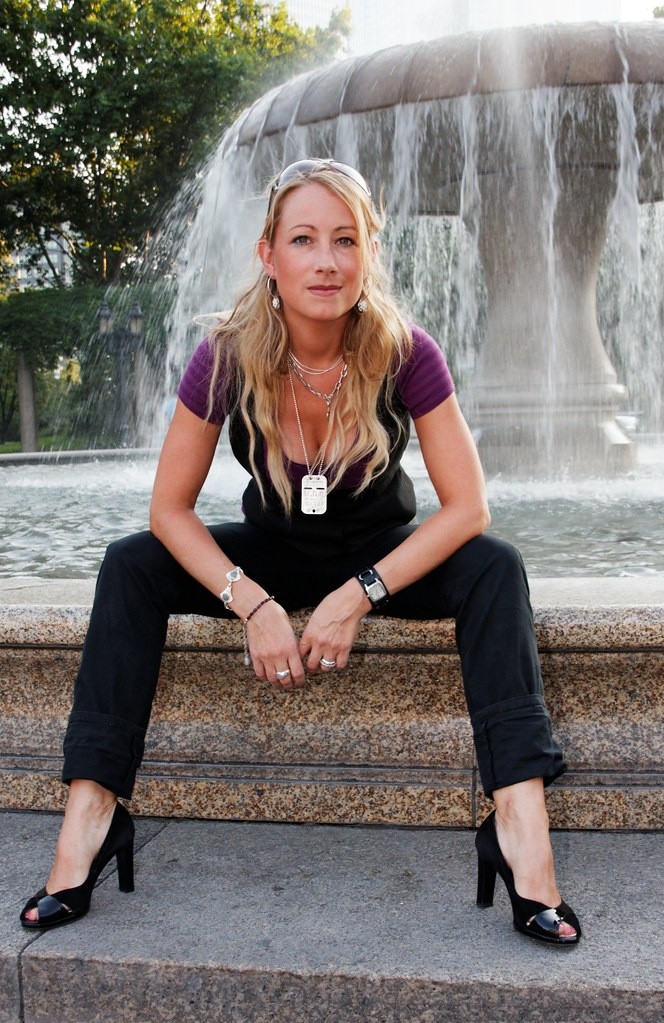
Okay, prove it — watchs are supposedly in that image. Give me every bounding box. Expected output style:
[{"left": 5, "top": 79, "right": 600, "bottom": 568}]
[{"left": 355, "top": 565, "right": 392, "bottom": 612}]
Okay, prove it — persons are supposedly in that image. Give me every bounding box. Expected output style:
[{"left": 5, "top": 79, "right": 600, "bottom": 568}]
[{"left": 19, "top": 158, "right": 583, "bottom": 950}]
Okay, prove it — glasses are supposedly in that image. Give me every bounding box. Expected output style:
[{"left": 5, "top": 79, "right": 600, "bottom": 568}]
[{"left": 266, "top": 158, "right": 372, "bottom": 214}]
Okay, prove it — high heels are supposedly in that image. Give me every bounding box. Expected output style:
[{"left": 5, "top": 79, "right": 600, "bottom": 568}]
[
  {"left": 20, "top": 802, "right": 137, "bottom": 930},
  {"left": 476, "top": 809, "right": 582, "bottom": 945}
]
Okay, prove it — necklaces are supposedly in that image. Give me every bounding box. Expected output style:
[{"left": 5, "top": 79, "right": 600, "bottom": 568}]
[
  {"left": 285, "top": 349, "right": 350, "bottom": 515},
  {"left": 285, "top": 349, "right": 352, "bottom": 419}
]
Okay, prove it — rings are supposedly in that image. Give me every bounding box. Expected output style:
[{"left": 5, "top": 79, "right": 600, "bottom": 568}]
[
  {"left": 320, "top": 658, "right": 336, "bottom": 667},
  {"left": 276, "top": 669, "right": 290, "bottom": 680}
]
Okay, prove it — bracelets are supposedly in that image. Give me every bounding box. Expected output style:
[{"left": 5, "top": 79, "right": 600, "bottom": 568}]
[
  {"left": 219, "top": 566, "right": 243, "bottom": 611},
  {"left": 243, "top": 595, "right": 275, "bottom": 666}
]
